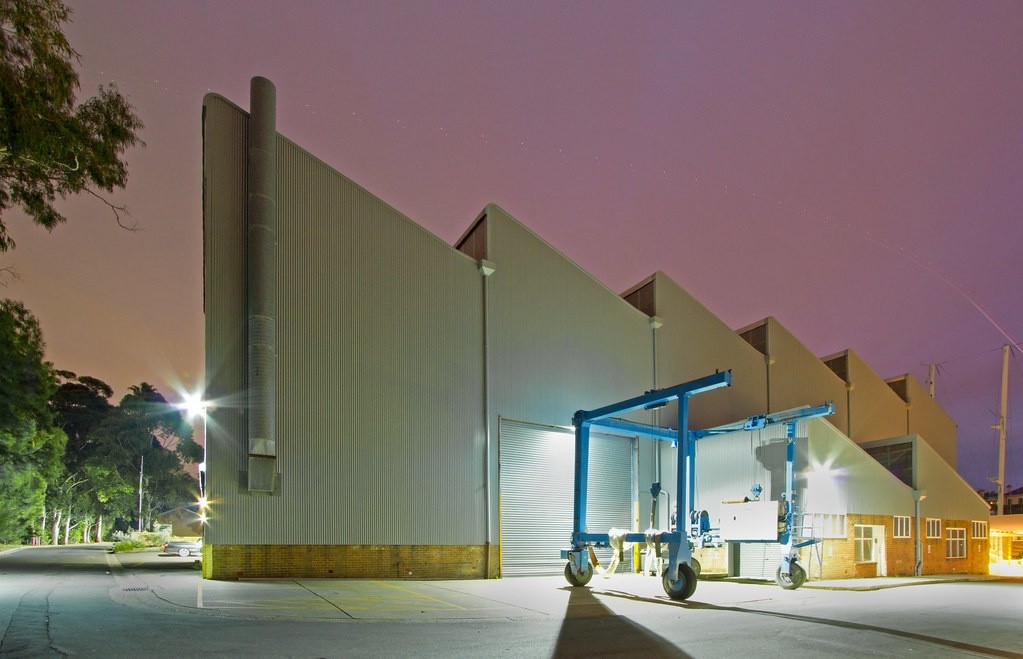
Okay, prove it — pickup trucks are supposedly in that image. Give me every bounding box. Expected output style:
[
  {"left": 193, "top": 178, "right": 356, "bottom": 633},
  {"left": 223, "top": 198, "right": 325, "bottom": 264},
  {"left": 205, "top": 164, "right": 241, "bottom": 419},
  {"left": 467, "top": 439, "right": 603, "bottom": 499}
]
[{"left": 163, "top": 538, "right": 204, "bottom": 558}]
